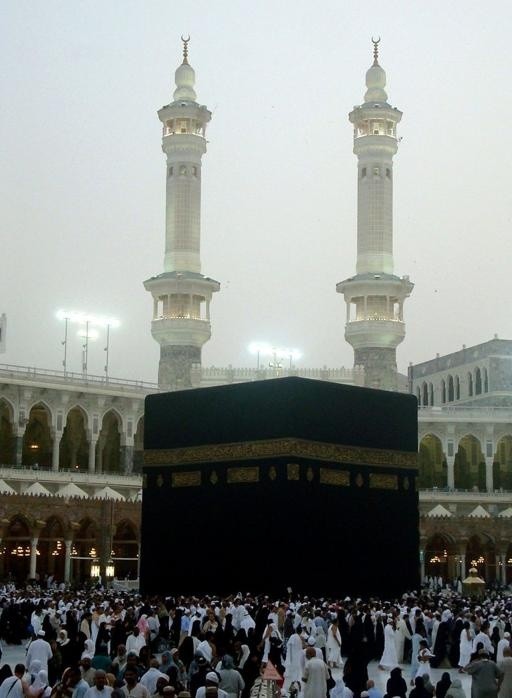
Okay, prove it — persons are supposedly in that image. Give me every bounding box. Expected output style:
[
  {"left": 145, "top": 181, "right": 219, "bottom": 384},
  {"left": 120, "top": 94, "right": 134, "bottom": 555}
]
[{"left": 1, "top": 574, "right": 511, "bottom": 698}]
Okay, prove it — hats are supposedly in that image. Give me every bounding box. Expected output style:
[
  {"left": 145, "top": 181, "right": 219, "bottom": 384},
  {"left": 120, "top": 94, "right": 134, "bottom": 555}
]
[
  {"left": 387, "top": 618, "right": 393, "bottom": 623},
  {"left": 185, "top": 609, "right": 191, "bottom": 613},
  {"left": 159, "top": 673, "right": 170, "bottom": 683},
  {"left": 308, "top": 637, "right": 316, "bottom": 645},
  {"left": 206, "top": 672, "right": 219, "bottom": 683},
  {"left": 504, "top": 632, "right": 510, "bottom": 638},
  {"left": 38, "top": 630, "right": 46, "bottom": 636}
]
